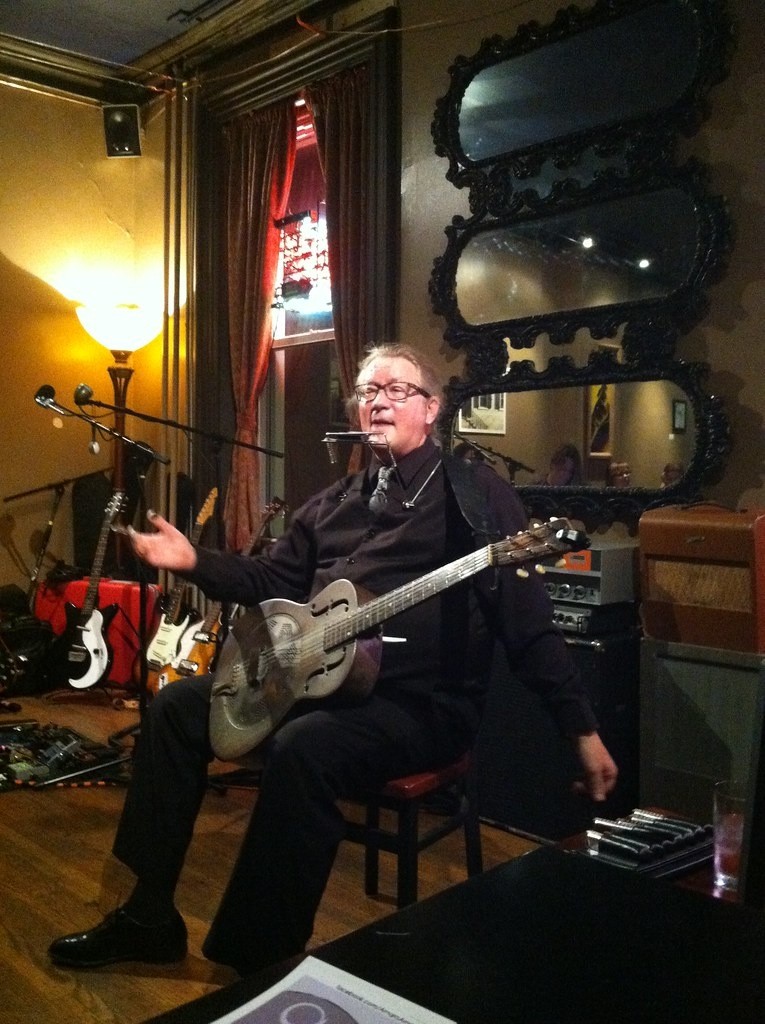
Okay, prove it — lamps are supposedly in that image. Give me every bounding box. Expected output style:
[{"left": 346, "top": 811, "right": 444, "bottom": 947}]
[{"left": 74, "top": 309, "right": 166, "bottom": 577}]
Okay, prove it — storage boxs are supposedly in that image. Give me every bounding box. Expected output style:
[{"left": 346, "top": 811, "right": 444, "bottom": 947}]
[{"left": 36, "top": 573, "right": 163, "bottom": 686}]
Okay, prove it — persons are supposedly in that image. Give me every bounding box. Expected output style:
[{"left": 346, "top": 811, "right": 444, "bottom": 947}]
[
  {"left": 455, "top": 438, "right": 682, "bottom": 487},
  {"left": 50, "top": 346, "right": 618, "bottom": 977}
]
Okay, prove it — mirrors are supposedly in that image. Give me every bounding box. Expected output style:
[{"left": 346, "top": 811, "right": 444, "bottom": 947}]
[
  {"left": 434, "top": 344, "right": 735, "bottom": 535},
  {"left": 431, "top": 1, "right": 739, "bottom": 197},
  {"left": 425, "top": 141, "right": 741, "bottom": 355}
]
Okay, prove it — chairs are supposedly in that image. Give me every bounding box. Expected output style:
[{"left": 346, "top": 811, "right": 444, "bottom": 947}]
[{"left": 335, "top": 575, "right": 501, "bottom": 913}]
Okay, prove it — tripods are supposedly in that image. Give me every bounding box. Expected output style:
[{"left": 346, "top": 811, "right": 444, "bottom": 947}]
[{"left": 32, "top": 407, "right": 228, "bottom": 794}]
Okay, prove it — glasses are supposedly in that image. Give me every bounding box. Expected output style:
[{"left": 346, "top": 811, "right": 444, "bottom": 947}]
[{"left": 352, "top": 381, "right": 431, "bottom": 403}]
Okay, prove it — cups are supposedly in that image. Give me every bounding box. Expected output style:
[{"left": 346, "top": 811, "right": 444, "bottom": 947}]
[{"left": 713, "top": 779, "right": 747, "bottom": 889}]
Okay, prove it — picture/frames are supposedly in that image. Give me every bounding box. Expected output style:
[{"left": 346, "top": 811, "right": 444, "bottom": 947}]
[{"left": 584, "top": 385, "right": 618, "bottom": 457}]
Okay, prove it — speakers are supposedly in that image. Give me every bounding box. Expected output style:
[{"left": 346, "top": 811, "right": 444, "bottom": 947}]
[
  {"left": 71, "top": 472, "right": 137, "bottom": 579},
  {"left": 103, "top": 103, "right": 142, "bottom": 159},
  {"left": 421, "top": 498, "right": 765, "bottom": 842}
]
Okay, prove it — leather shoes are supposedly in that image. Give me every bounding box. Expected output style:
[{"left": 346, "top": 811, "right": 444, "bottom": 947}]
[{"left": 48, "top": 892, "right": 188, "bottom": 969}]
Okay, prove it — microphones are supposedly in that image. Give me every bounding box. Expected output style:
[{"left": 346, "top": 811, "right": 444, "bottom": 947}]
[
  {"left": 74, "top": 385, "right": 93, "bottom": 405},
  {"left": 35, "top": 385, "right": 55, "bottom": 402}
]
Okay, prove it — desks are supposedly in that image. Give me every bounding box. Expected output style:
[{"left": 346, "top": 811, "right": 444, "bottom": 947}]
[{"left": 140, "top": 839, "right": 765, "bottom": 1024}]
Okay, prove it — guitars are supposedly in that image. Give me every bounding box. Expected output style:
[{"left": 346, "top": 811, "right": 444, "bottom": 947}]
[
  {"left": 133, "top": 485, "right": 202, "bottom": 690},
  {"left": 41, "top": 491, "right": 129, "bottom": 691},
  {"left": 210, "top": 513, "right": 593, "bottom": 767},
  {"left": 152, "top": 497, "right": 286, "bottom": 699}
]
